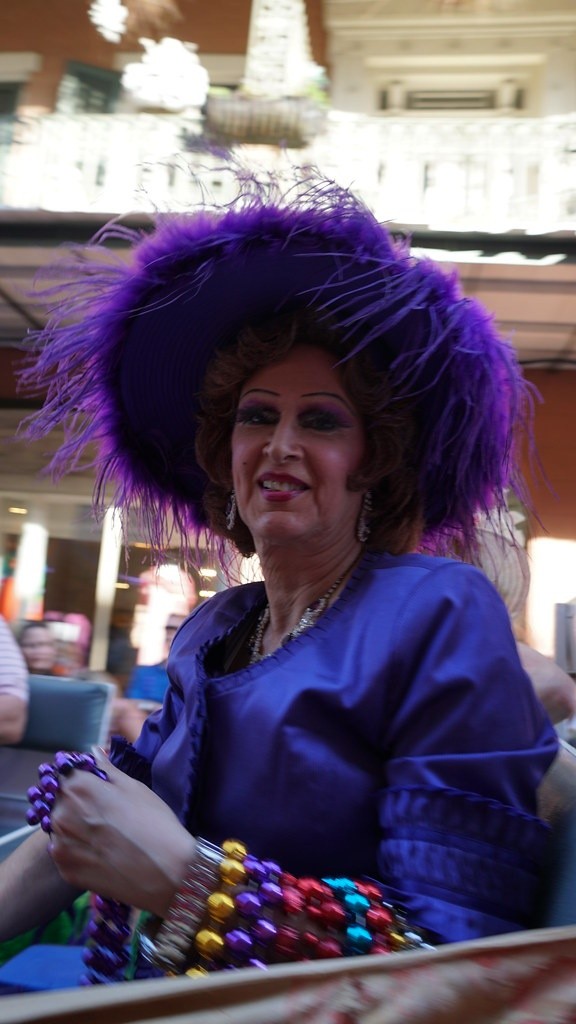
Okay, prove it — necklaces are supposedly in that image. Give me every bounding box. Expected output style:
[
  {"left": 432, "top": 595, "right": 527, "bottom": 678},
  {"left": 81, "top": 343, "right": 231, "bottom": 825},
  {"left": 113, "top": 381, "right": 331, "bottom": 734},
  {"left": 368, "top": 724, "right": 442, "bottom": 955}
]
[
  {"left": 28, "top": 749, "right": 133, "bottom": 984},
  {"left": 246, "top": 577, "right": 343, "bottom": 665}
]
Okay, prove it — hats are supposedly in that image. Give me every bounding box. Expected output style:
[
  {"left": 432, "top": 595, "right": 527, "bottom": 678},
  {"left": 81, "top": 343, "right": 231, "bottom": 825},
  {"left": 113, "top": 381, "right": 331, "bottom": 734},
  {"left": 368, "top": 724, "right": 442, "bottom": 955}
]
[{"left": 19, "top": 136, "right": 557, "bottom": 592}]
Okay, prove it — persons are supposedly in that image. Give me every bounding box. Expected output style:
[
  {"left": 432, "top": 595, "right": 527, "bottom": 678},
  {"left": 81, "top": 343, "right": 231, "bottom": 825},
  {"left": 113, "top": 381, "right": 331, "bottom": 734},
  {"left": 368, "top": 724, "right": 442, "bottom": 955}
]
[
  {"left": 441, "top": 509, "right": 576, "bottom": 746},
  {"left": 125, "top": 615, "right": 187, "bottom": 703},
  {"left": 19, "top": 623, "right": 58, "bottom": 675},
  {"left": 0, "top": 339, "right": 558, "bottom": 983},
  {"left": 0, "top": 616, "right": 30, "bottom": 743}
]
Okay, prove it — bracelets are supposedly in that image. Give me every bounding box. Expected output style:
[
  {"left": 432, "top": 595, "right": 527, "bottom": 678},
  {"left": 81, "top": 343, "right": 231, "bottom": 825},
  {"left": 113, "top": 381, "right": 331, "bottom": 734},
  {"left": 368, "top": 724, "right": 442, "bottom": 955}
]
[{"left": 134, "top": 838, "right": 433, "bottom": 980}]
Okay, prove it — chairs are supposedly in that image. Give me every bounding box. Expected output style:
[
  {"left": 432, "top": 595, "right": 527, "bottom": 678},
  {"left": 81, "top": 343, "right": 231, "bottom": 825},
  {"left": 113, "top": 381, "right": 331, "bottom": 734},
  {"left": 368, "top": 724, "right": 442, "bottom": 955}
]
[{"left": 0, "top": 674, "right": 116, "bottom": 822}]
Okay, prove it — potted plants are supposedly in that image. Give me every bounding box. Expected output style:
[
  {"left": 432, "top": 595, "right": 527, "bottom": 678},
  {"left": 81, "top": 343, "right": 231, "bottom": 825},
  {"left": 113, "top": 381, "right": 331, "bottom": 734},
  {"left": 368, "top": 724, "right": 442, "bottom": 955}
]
[{"left": 206, "top": 86, "right": 331, "bottom": 150}]
